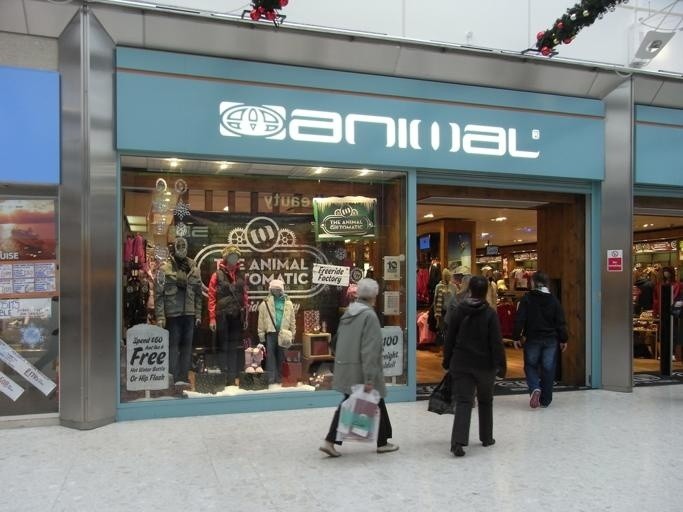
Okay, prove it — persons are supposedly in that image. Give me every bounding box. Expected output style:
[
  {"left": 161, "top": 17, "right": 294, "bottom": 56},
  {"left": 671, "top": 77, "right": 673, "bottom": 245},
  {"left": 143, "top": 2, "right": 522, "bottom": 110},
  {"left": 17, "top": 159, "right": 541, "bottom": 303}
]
[
  {"left": 482, "top": 266, "right": 499, "bottom": 313},
  {"left": 258, "top": 280, "right": 297, "bottom": 384},
  {"left": 153, "top": 239, "right": 203, "bottom": 391},
  {"left": 319, "top": 277, "right": 399, "bottom": 458},
  {"left": 512, "top": 269, "right": 568, "bottom": 409},
  {"left": 433, "top": 269, "right": 458, "bottom": 355},
  {"left": 444, "top": 276, "right": 508, "bottom": 456},
  {"left": 651, "top": 266, "right": 681, "bottom": 361},
  {"left": 206, "top": 244, "right": 249, "bottom": 391}
]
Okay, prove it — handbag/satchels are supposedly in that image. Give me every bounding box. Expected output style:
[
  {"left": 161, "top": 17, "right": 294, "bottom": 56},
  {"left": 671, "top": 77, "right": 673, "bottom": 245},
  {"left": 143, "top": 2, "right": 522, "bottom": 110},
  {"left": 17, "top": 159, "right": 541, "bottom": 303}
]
[
  {"left": 241, "top": 308, "right": 245, "bottom": 325},
  {"left": 428, "top": 371, "right": 475, "bottom": 414},
  {"left": 278, "top": 330, "right": 292, "bottom": 349}
]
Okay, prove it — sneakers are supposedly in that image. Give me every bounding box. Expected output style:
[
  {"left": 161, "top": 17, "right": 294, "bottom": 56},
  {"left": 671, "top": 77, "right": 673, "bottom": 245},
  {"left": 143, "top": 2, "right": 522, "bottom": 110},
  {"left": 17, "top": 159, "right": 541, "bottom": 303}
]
[
  {"left": 320, "top": 441, "right": 341, "bottom": 456},
  {"left": 377, "top": 442, "right": 398, "bottom": 453},
  {"left": 530, "top": 389, "right": 541, "bottom": 408}
]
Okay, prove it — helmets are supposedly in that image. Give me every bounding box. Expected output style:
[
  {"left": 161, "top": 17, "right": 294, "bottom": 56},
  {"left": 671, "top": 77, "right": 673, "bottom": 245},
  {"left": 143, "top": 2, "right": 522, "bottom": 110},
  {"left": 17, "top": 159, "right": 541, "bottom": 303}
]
[{"left": 169, "top": 238, "right": 188, "bottom": 258}]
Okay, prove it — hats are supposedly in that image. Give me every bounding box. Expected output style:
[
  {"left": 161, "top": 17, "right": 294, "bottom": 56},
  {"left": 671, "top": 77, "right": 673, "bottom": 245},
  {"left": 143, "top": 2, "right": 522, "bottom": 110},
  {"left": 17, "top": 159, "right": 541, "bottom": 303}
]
[
  {"left": 269, "top": 280, "right": 284, "bottom": 295},
  {"left": 357, "top": 278, "right": 379, "bottom": 298},
  {"left": 222, "top": 245, "right": 241, "bottom": 259}
]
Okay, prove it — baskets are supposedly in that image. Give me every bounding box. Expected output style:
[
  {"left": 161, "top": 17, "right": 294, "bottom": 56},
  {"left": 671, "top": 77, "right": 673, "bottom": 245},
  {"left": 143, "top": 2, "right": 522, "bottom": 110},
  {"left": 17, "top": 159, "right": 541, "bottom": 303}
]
[
  {"left": 195, "top": 372, "right": 224, "bottom": 393},
  {"left": 239, "top": 371, "right": 268, "bottom": 390}
]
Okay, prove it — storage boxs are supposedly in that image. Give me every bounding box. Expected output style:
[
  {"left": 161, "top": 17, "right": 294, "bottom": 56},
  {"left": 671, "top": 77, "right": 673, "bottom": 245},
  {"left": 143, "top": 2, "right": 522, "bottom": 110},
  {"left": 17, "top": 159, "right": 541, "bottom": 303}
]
[
  {"left": 311, "top": 340, "right": 328, "bottom": 355},
  {"left": 238, "top": 369, "right": 270, "bottom": 391},
  {"left": 193, "top": 370, "right": 227, "bottom": 394}
]
